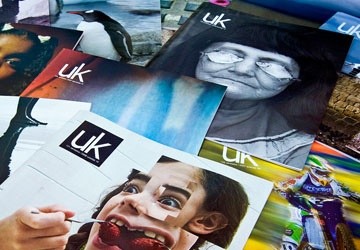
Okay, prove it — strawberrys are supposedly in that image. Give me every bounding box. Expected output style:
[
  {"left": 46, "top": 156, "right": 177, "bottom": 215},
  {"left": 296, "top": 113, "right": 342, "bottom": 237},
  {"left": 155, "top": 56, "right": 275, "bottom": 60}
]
[
  {"left": 99, "top": 221, "right": 121, "bottom": 245},
  {"left": 126, "top": 237, "right": 162, "bottom": 250}
]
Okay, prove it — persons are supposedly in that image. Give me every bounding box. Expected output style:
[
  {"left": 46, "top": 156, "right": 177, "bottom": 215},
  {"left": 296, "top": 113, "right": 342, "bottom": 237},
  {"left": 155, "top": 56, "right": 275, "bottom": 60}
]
[
  {"left": 165, "top": 20, "right": 320, "bottom": 172},
  {"left": 0, "top": 155, "right": 250, "bottom": 250},
  {"left": 271, "top": 154, "right": 360, "bottom": 250},
  {"left": 1, "top": 23, "right": 58, "bottom": 98}
]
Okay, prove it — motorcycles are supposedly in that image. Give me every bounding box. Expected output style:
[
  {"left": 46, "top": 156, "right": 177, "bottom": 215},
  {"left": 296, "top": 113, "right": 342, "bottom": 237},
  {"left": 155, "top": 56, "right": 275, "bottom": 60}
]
[{"left": 287, "top": 190, "right": 356, "bottom": 250}]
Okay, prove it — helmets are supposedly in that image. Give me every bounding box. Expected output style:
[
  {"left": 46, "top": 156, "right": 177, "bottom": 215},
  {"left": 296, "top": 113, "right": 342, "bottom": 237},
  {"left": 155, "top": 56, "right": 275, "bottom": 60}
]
[{"left": 310, "top": 162, "right": 334, "bottom": 184}]
